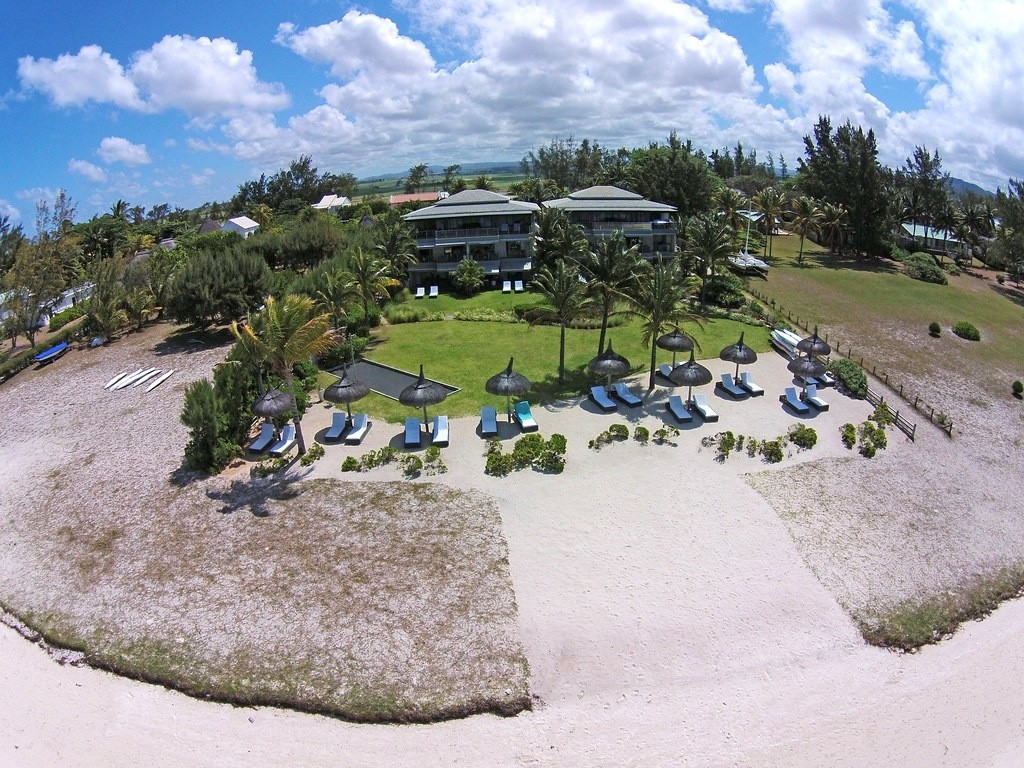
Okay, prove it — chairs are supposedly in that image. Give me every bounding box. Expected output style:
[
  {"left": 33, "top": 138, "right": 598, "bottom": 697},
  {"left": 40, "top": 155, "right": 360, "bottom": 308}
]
[
  {"left": 590, "top": 386, "right": 616, "bottom": 407},
  {"left": 415, "top": 287, "right": 425, "bottom": 297},
  {"left": 658, "top": 364, "right": 672, "bottom": 376},
  {"left": 800, "top": 376, "right": 819, "bottom": 384},
  {"left": 740, "top": 372, "right": 764, "bottom": 392},
  {"left": 269, "top": 426, "right": 295, "bottom": 454},
  {"left": 784, "top": 387, "right": 809, "bottom": 410},
  {"left": 721, "top": 373, "right": 746, "bottom": 395},
  {"left": 502, "top": 281, "right": 511, "bottom": 292},
  {"left": 480, "top": 406, "right": 497, "bottom": 433},
  {"left": 433, "top": 415, "right": 448, "bottom": 442},
  {"left": 429, "top": 286, "right": 438, "bottom": 297},
  {"left": 615, "top": 382, "right": 642, "bottom": 404},
  {"left": 249, "top": 424, "right": 274, "bottom": 450},
  {"left": 693, "top": 394, "right": 718, "bottom": 418},
  {"left": 669, "top": 395, "right": 692, "bottom": 419},
  {"left": 345, "top": 413, "right": 367, "bottom": 440},
  {"left": 805, "top": 384, "right": 829, "bottom": 406},
  {"left": 515, "top": 281, "right": 523, "bottom": 291},
  {"left": 819, "top": 374, "right": 835, "bottom": 383},
  {"left": 405, "top": 418, "right": 420, "bottom": 443},
  {"left": 324, "top": 413, "right": 346, "bottom": 438},
  {"left": 514, "top": 401, "right": 538, "bottom": 428}
]
